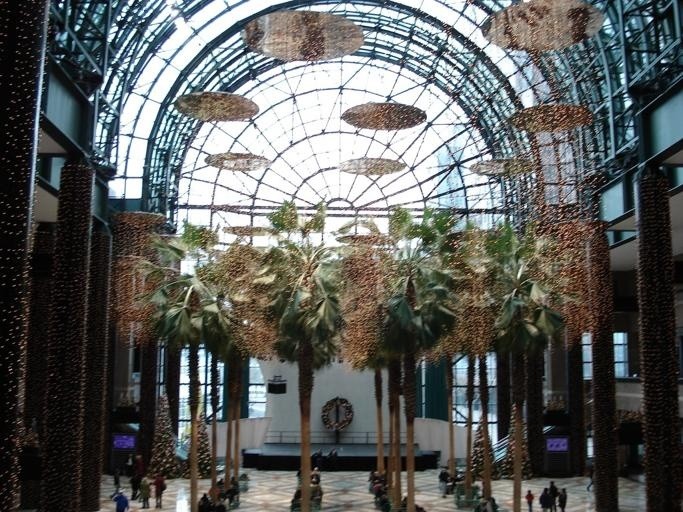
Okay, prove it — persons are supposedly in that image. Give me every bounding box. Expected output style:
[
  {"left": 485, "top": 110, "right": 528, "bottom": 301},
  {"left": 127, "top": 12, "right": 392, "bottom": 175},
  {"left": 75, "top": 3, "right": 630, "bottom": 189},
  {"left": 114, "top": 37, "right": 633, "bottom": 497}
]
[
  {"left": 367, "top": 470, "right": 425, "bottom": 511},
  {"left": 108, "top": 455, "right": 168, "bottom": 511},
  {"left": 437, "top": 465, "right": 499, "bottom": 512},
  {"left": 197, "top": 476, "right": 240, "bottom": 511},
  {"left": 525, "top": 481, "right": 567, "bottom": 511},
  {"left": 289, "top": 467, "right": 324, "bottom": 511},
  {"left": 587, "top": 466, "right": 595, "bottom": 491}
]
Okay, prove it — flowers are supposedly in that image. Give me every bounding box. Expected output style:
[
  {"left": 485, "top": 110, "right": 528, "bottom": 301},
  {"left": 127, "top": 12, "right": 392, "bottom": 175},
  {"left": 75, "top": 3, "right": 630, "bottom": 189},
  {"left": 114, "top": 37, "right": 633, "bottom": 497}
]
[{"left": 321, "top": 397, "right": 354, "bottom": 431}]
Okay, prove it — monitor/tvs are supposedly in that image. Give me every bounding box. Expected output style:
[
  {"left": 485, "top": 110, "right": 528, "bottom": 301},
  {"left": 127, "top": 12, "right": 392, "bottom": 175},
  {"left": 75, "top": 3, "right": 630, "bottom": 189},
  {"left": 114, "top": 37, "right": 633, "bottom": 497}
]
[
  {"left": 112, "top": 434, "right": 136, "bottom": 449},
  {"left": 545, "top": 437, "right": 568, "bottom": 452}
]
